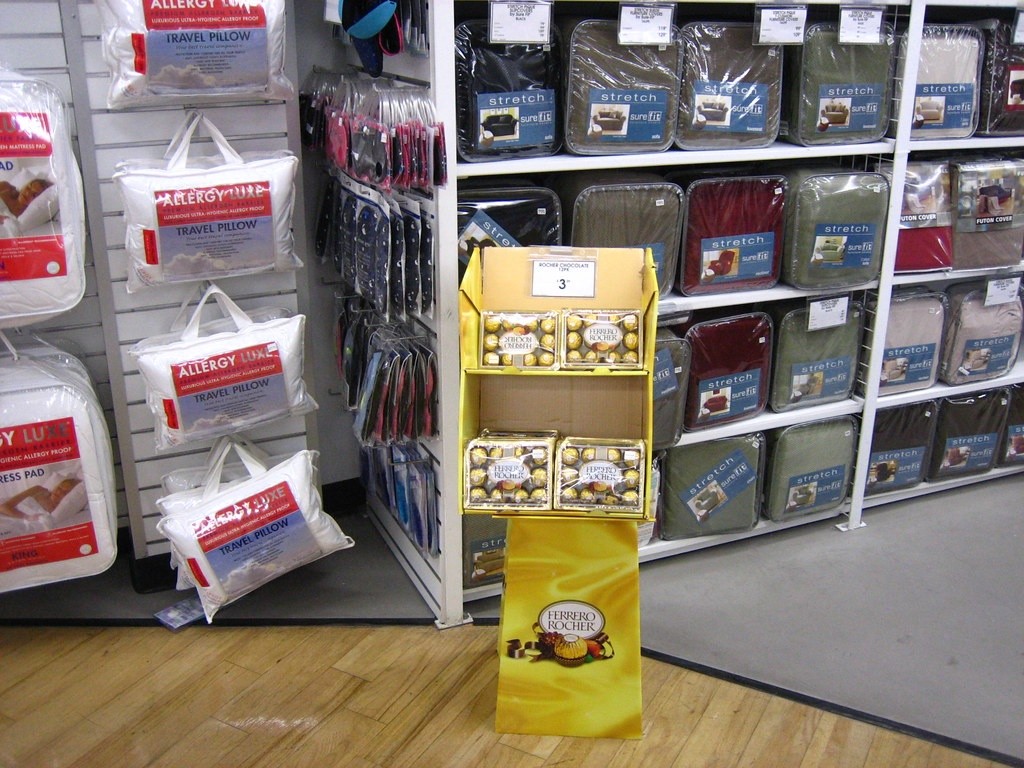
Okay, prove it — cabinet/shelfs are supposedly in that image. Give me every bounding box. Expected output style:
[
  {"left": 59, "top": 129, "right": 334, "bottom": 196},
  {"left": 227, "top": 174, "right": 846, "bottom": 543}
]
[
  {"left": 455, "top": 242, "right": 661, "bottom": 529},
  {"left": 343, "top": 0, "right": 1024, "bottom": 632}
]
